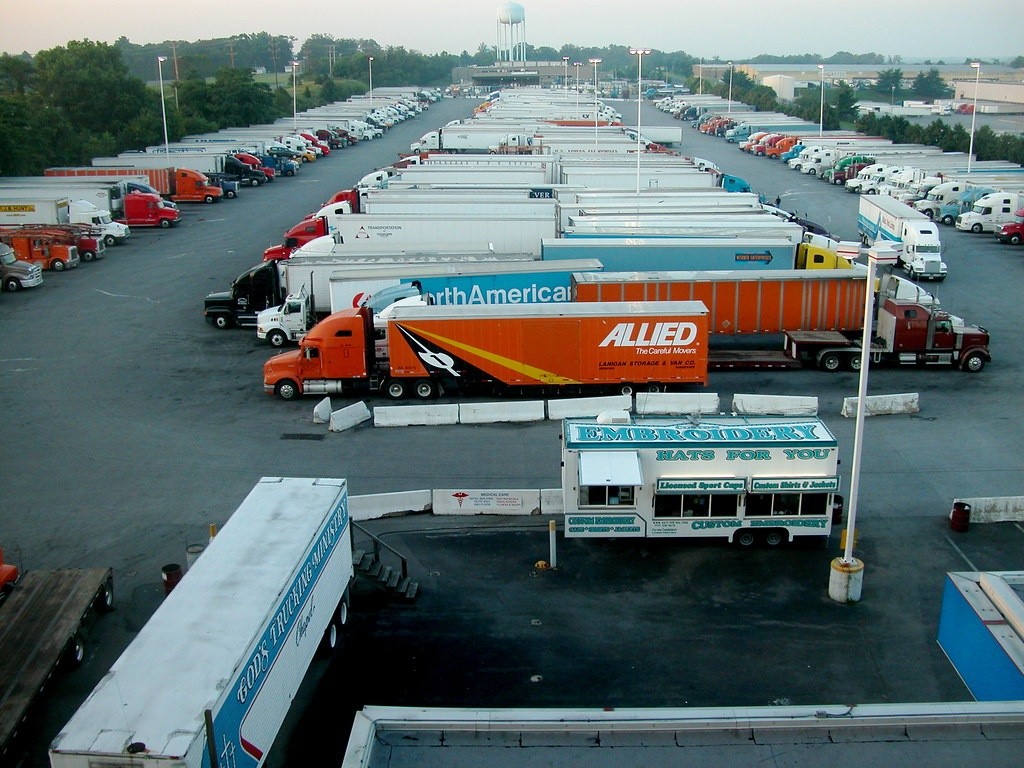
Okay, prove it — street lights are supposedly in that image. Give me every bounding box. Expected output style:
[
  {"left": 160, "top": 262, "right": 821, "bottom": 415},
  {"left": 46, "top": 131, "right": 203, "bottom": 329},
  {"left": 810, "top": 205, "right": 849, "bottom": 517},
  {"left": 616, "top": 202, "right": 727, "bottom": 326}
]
[
  {"left": 572, "top": 62, "right": 582, "bottom": 111},
  {"left": 816, "top": 64, "right": 826, "bottom": 137},
  {"left": 629, "top": 49, "right": 652, "bottom": 193},
  {"left": 562, "top": 56, "right": 571, "bottom": 98},
  {"left": 157, "top": 56, "right": 171, "bottom": 167},
  {"left": 968, "top": 63, "right": 981, "bottom": 174},
  {"left": 368, "top": 57, "right": 374, "bottom": 108},
  {"left": 890, "top": 85, "right": 896, "bottom": 117},
  {"left": 727, "top": 62, "right": 734, "bottom": 111},
  {"left": 588, "top": 57, "right": 605, "bottom": 151},
  {"left": 292, "top": 61, "right": 299, "bottom": 134},
  {"left": 827, "top": 246, "right": 898, "bottom": 603}
]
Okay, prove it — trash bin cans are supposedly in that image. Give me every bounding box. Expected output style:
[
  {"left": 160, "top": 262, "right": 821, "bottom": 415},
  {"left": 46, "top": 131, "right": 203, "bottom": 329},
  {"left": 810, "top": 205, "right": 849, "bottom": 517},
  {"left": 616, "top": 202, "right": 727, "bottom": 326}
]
[
  {"left": 832, "top": 494, "right": 844, "bottom": 525},
  {"left": 186, "top": 544, "right": 205, "bottom": 569},
  {"left": 951, "top": 502, "right": 971, "bottom": 531},
  {"left": 162, "top": 564, "right": 182, "bottom": 597}
]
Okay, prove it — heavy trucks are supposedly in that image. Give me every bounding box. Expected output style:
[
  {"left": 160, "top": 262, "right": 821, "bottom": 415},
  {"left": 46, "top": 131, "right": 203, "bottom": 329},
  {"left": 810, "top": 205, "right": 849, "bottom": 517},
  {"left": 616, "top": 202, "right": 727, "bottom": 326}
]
[
  {"left": 263, "top": 296, "right": 709, "bottom": 399},
  {"left": 0, "top": 85, "right": 1024, "bottom": 373}
]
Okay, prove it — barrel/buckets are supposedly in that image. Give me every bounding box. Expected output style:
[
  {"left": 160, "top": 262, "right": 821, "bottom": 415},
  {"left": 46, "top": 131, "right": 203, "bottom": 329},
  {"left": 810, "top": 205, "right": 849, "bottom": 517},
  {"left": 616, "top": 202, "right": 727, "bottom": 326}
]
[
  {"left": 161, "top": 563, "right": 183, "bottom": 597},
  {"left": 186, "top": 543, "right": 206, "bottom": 570}
]
